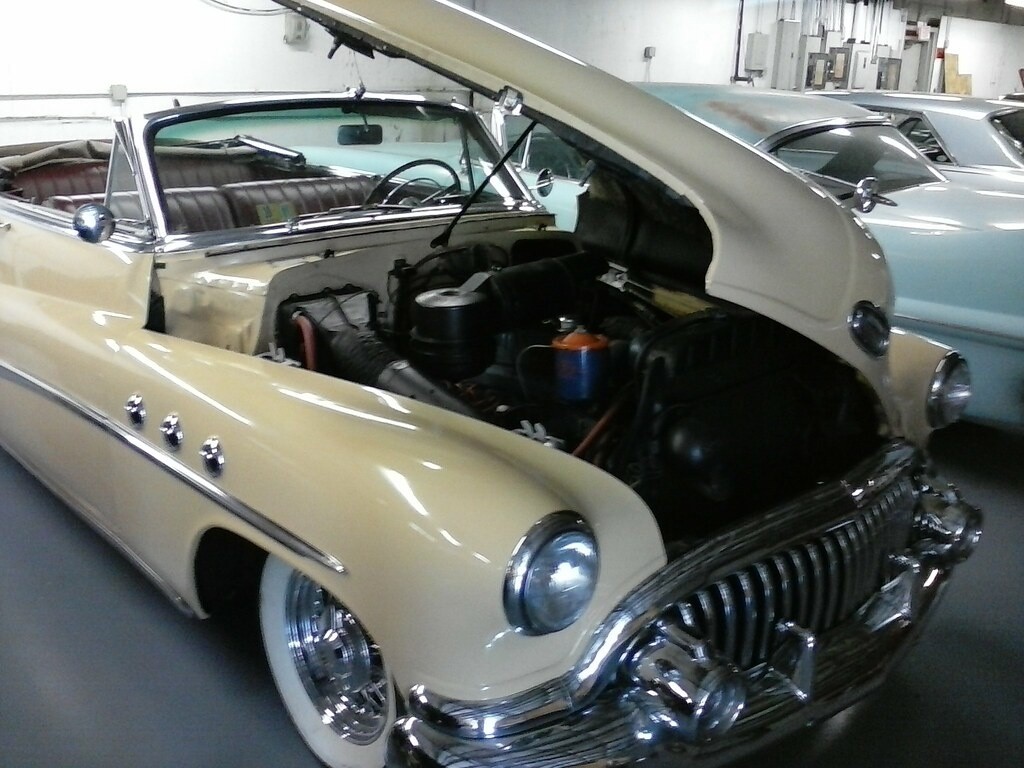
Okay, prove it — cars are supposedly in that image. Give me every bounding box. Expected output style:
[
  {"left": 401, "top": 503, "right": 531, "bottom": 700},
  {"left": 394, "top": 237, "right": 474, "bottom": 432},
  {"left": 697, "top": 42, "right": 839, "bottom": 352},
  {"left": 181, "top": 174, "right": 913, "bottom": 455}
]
[
  {"left": 287, "top": 80, "right": 1023, "bottom": 437},
  {"left": 807, "top": 90, "right": 1023, "bottom": 173},
  {"left": 0, "top": 2, "right": 983, "bottom": 763}
]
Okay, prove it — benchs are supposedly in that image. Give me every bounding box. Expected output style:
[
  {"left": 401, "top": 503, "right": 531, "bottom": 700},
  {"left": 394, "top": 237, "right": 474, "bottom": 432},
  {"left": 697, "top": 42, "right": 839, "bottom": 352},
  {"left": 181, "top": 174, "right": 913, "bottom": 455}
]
[{"left": 13, "top": 150, "right": 385, "bottom": 239}]
